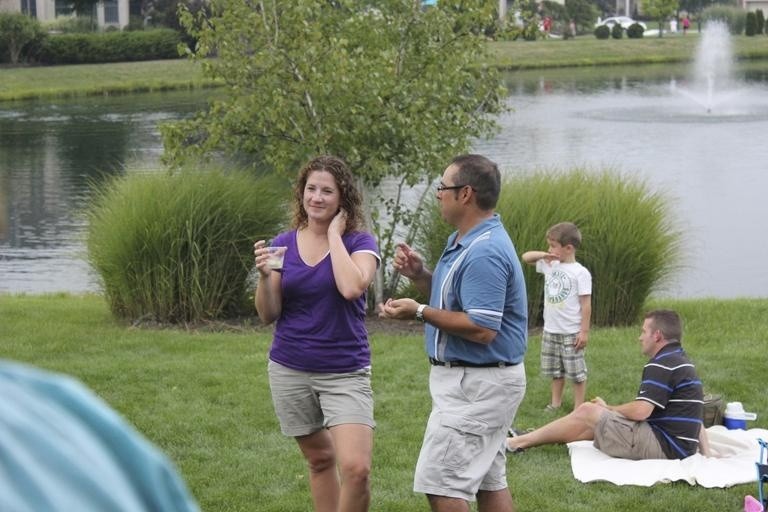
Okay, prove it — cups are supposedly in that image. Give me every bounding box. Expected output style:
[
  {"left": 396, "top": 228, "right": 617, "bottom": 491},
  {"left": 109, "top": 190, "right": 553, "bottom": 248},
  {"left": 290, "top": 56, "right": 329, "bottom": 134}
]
[{"left": 264, "top": 247, "right": 285, "bottom": 270}]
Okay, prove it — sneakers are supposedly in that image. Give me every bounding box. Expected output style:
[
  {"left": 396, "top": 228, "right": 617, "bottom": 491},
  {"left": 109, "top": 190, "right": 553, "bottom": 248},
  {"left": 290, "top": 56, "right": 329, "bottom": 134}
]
[{"left": 503, "top": 426, "right": 528, "bottom": 455}]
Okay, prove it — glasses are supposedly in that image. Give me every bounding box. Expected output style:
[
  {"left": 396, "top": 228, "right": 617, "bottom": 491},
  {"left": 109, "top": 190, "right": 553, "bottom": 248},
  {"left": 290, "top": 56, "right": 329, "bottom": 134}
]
[{"left": 437, "top": 185, "right": 477, "bottom": 194}]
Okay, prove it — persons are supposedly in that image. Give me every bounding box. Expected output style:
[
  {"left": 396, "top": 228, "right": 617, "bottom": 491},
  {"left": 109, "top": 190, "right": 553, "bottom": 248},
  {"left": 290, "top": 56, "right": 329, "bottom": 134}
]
[
  {"left": 504, "top": 307, "right": 711, "bottom": 461},
  {"left": 253, "top": 153, "right": 383, "bottom": 510},
  {"left": 377, "top": 152, "right": 529, "bottom": 512},
  {"left": 543, "top": 14, "right": 551, "bottom": 41},
  {"left": 669, "top": 15, "right": 677, "bottom": 36},
  {"left": 567, "top": 18, "right": 576, "bottom": 40},
  {"left": 520, "top": 221, "right": 594, "bottom": 414},
  {"left": 681, "top": 15, "right": 690, "bottom": 35}
]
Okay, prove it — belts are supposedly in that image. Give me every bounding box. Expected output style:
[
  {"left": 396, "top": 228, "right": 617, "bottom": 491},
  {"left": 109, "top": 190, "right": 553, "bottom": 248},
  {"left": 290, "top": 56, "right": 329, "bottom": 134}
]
[{"left": 428, "top": 356, "right": 518, "bottom": 367}]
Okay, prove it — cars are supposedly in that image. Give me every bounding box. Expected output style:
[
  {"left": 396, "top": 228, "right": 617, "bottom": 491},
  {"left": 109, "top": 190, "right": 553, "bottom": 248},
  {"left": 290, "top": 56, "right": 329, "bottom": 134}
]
[{"left": 594, "top": 16, "right": 647, "bottom": 32}]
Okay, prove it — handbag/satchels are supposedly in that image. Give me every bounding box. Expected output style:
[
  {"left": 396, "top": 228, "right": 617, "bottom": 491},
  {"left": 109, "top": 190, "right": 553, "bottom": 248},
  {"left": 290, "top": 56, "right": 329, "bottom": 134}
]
[{"left": 704, "top": 393, "right": 724, "bottom": 426}]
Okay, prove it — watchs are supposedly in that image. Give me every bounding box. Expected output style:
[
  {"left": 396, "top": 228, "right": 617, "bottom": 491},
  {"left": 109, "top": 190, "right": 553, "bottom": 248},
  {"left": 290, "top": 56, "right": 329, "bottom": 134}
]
[{"left": 413, "top": 304, "right": 428, "bottom": 325}]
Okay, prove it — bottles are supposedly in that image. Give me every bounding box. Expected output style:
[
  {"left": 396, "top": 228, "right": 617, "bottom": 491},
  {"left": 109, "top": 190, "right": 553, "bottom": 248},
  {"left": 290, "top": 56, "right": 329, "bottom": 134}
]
[{"left": 724, "top": 402, "right": 757, "bottom": 430}]
